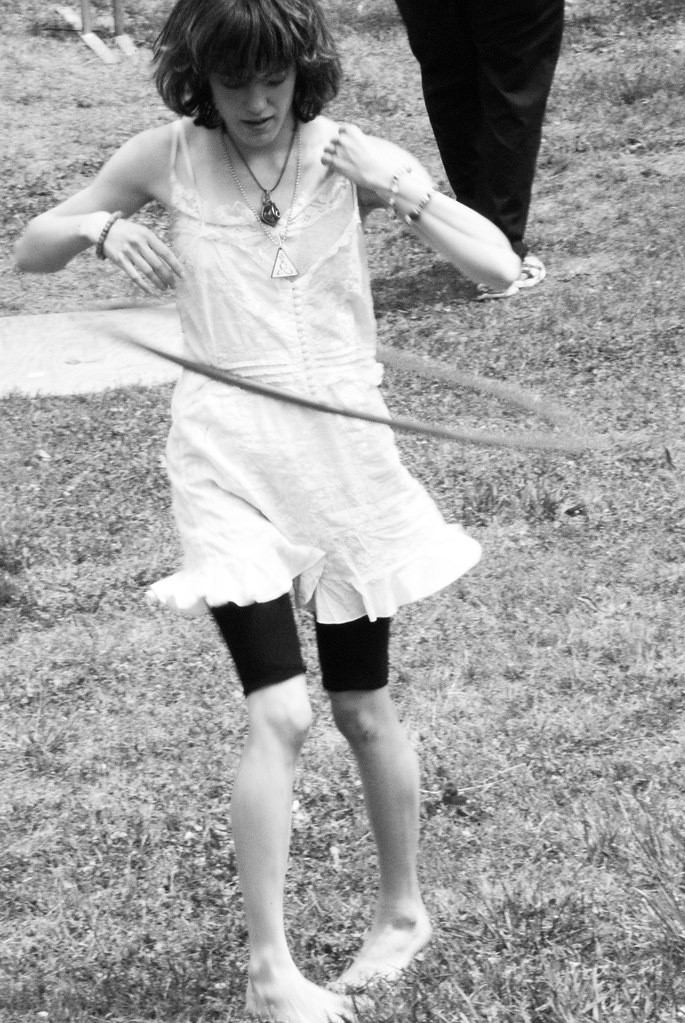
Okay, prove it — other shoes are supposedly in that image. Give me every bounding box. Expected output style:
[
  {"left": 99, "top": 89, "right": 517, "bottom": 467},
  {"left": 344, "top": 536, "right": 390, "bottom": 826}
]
[{"left": 477, "top": 255, "right": 546, "bottom": 300}]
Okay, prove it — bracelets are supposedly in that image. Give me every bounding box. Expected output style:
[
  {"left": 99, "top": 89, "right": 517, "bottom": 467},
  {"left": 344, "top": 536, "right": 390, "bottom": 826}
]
[
  {"left": 96, "top": 211, "right": 123, "bottom": 259},
  {"left": 389, "top": 169, "right": 410, "bottom": 218},
  {"left": 405, "top": 185, "right": 438, "bottom": 226}
]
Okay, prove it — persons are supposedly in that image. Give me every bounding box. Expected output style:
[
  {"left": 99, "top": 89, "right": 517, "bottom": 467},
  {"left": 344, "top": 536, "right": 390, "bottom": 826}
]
[
  {"left": 397, "top": 0, "right": 566, "bottom": 296},
  {"left": 17, "top": 0, "right": 524, "bottom": 1023}
]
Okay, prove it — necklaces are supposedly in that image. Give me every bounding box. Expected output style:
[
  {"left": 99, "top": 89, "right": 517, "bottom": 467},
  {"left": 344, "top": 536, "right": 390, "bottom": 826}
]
[
  {"left": 216, "top": 126, "right": 300, "bottom": 277},
  {"left": 226, "top": 119, "right": 297, "bottom": 227}
]
